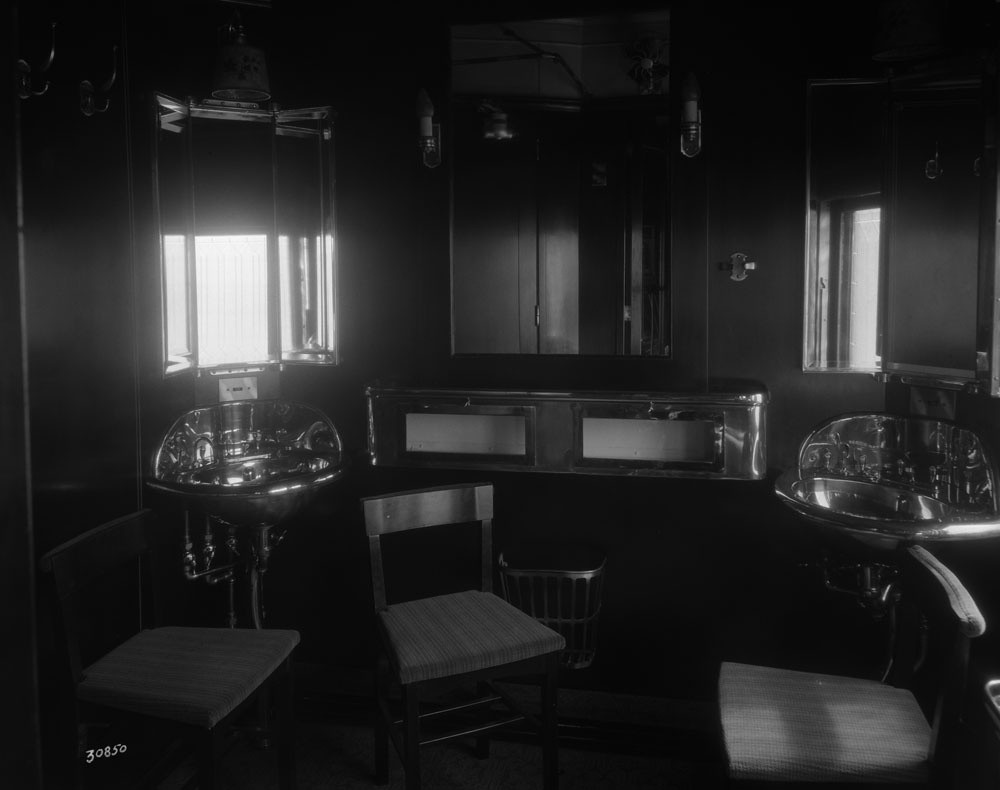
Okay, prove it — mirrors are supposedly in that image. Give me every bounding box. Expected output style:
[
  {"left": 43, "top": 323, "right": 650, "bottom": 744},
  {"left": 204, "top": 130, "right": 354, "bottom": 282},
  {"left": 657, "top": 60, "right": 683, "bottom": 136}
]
[
  {"left": 151, "top": 91, "right": 341, "bottom": 379},
  {"left": 802, "top": 71, "right": 1000, "bottom": 396},
  {"left": 447, "top": 6, "right": 675, "bottom": 364}
]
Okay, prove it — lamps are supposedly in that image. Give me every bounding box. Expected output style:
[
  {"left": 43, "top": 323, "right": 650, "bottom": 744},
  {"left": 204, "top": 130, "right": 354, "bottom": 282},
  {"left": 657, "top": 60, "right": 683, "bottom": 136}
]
[
  {"left": 680, "top": 72, "right": 701, "bottom": 159},
  {"left": 417, "top": 86, "right": 441, "bottom": 169}
]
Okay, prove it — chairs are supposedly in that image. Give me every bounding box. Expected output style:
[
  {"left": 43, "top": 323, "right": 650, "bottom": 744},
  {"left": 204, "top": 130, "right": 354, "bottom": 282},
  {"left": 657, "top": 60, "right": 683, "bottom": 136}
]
[
  {"left": 718, "top": 541, "right": 988, "bottom": 790},
  {"left": 36, "top": 506, "right": 303, "bottom": 790},
  {"left": 360, "top": 479, "right": 568, "bottom": 790}
]
